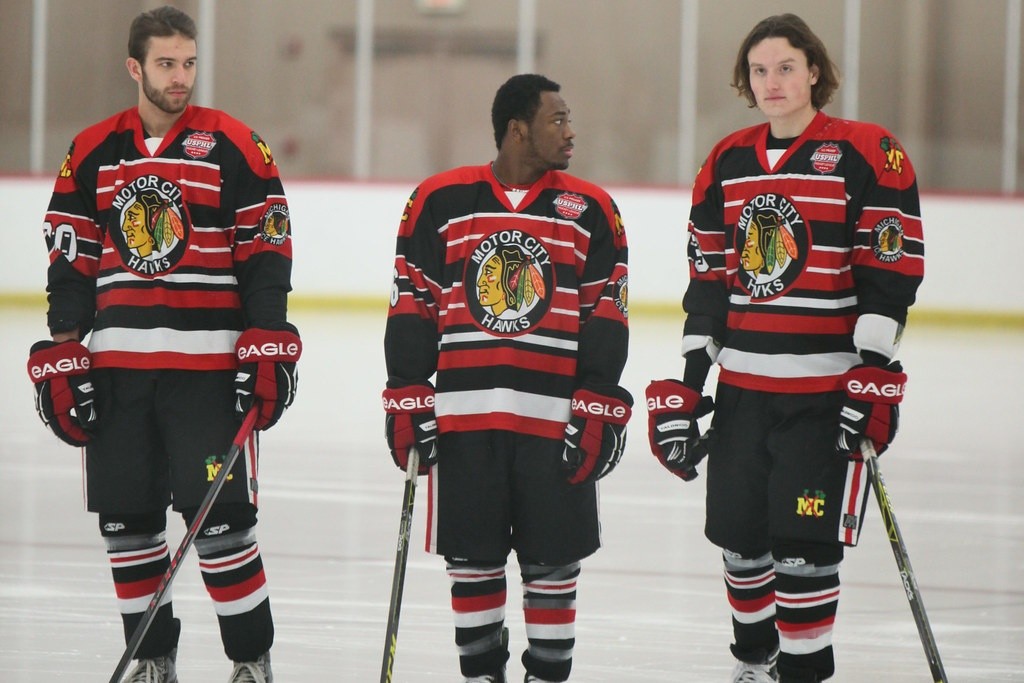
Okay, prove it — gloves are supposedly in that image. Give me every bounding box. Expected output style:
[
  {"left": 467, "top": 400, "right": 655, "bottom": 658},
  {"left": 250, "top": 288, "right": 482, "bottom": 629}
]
[
  {"left": 560, "top": 385, "right": 633, "bottom": 487},
  {"left": 27, "top": 339, "right": 98, "bottom": 447},
  {"left": 383, "top": 377, "right": 437, "bottom": 476},
  {"left": 832, "top": 361, "right": 907, "bottom": 462},
  {"left": 235, "top": 323, "right": 301, "bottom": 432},
  {"left": 645, "top": 379, "right": 718, "bottom": 481}
]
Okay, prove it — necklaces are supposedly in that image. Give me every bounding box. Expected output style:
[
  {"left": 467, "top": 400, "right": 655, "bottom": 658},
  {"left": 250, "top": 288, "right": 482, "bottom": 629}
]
[{"left": 491, "top": 163, "right": 529, "bottom": 192}]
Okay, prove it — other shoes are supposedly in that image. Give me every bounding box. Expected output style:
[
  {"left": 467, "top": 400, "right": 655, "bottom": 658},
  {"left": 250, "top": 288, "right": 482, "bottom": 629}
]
[
  {"left": 229, "top": 651, "right": 274, "bottom": 683},
  {"left": 730, "top": 643, "right": 780, "bottom": 683},
  {"left": 121, "top": 616, "right": 181, "bottom": 683}
]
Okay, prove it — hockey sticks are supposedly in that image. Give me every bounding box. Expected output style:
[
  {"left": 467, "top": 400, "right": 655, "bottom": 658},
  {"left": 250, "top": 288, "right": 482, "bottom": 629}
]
[
  {"left": 859, "top": 437, "right": 950, "bottom": 683},
  {"left": 110, "top": 399, "right": 258, "bottom": 683},
  {"left": 380, "top": 447, "right": 421, "bottom": 682}
]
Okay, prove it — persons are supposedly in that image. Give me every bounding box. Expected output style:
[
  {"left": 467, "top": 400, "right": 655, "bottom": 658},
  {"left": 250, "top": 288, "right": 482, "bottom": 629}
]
[
  {"left": 645, "top": 13, "right": 924, "bottom": 683},
  {"left": 27, "top": 5, "right": 303, "bottom": 683},
  {"left": 381, "top": 75, "right": 634, "bottom": 683}
]
